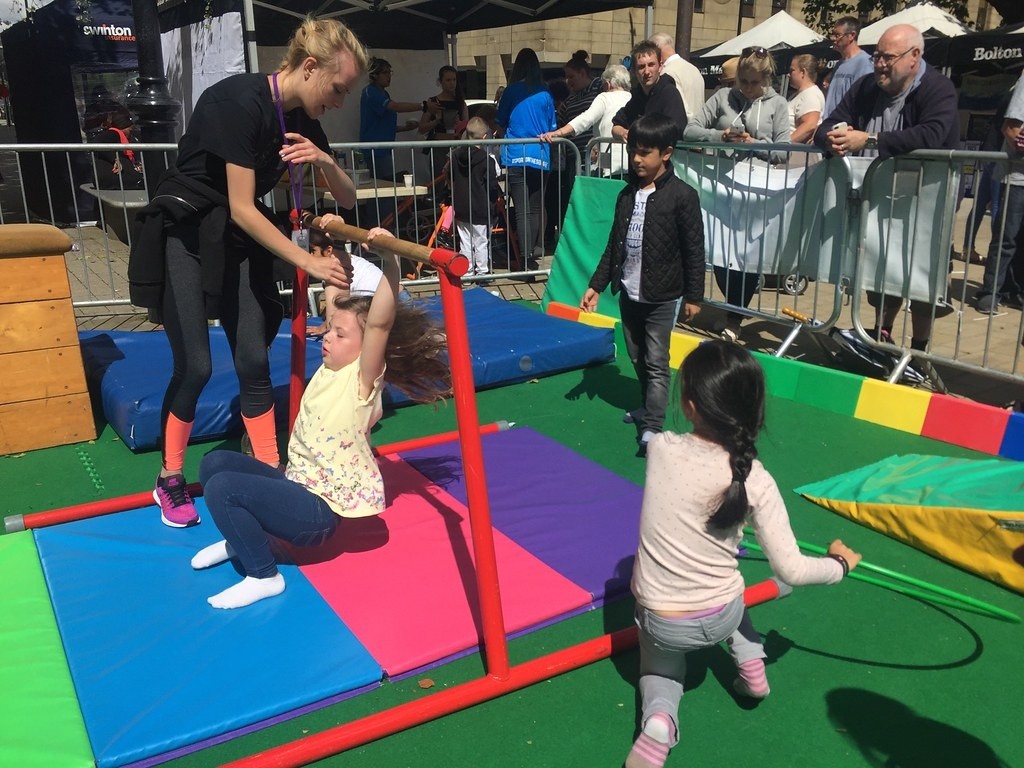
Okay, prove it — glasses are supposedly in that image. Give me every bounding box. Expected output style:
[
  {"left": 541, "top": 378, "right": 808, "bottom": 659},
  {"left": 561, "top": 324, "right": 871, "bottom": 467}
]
[
  {"left": 868, "top": 47, "right": 913, "bottom": 66},
  {"left": 381, "top": 71, "right": 393, "bottom": 76},
  {"left": 743, "top": 47, "right": 768, "bottom": 59},
  {"left": 829, "top": 32, "right": 850, "bottom": 38}
]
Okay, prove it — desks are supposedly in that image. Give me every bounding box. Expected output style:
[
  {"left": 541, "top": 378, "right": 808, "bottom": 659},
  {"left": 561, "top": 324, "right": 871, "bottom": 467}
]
[
  {"left": 284, "top": 174, "right": 428, "bottom": 249},
  {"left": 80, "top": 182, "right": 149, "bottom": 242}
]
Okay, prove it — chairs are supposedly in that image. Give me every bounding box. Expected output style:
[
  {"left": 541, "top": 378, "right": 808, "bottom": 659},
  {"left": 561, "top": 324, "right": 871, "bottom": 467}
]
[{"left": 469, "top": 198, "right": 522, "bottom": 274}]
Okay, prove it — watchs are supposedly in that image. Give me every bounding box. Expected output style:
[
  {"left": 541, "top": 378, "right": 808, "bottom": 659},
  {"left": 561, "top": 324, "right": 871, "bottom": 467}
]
[{"left": 865, "top": 132, "right": 878, "bottom": 149}]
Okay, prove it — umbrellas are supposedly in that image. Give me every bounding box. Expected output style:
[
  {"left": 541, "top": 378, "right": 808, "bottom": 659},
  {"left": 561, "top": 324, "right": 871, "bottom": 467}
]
[
  {"left": 699, "top": 9, "right": 829, "bottom": 58},
  {"left": 857, "top": 0, "right": 979, "bottom": 46},
  {"left": 782, "top": 306, "right": 932, "bottom": 385}
]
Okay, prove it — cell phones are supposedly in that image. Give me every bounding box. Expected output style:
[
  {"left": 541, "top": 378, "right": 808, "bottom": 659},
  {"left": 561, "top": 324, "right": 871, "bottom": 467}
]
[
  {"left": 730, "top": 124, "right": 745, "bottom": 133},
  {"left": 831, "top": 121, "right": 848, "bottom": 132}
]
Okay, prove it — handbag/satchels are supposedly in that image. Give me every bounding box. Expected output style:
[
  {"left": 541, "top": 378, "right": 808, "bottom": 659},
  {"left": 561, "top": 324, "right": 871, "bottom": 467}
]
[{"left": 417, "top": 97, "right": 437, "bottom": 155}]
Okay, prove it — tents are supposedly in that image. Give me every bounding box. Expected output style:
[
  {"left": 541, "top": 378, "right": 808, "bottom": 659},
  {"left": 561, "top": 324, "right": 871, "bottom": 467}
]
[{"left": 0, "top": 0, "right": 655, "bottom": 228}]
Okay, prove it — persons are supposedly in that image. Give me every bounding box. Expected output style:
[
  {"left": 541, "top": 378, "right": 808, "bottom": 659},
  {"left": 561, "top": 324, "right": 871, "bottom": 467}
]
[
  {"left": 418, "top": 65, "right": 469, "bottom": 193},
  {"left": 974, "top": 68, "right": 1024, "bottom": 315},
  {"left": 814, "top": 24, "right": 966, "bottom": 380},
  {"left": 960, "top": 91, "right": 1013, "bottom": 265},
  {"left": 82, "top": 85, "right": 139, "bottom": 191},
  {"left": 579, "top": 114, "right": 706, "bottom": 446},
  {"left": 191, "top": 213, "right": 454, "bottom": 609},
  {"left": 625, "top": 338, "right": 863, "bottom": 768},
  {"left": 494, "top": 31, "right": 825, "bottom": 341},
  {"left": 127, "top": 11, "right": 369, "bottom": 528},
  {"left": 360, "top": 56, "right": 445, "bottom": 225},
  {"left": 447, "top": 117, "right": 504, "bottom": 287},
  {"left": 822, "top": 16, "right": 875, "bottom": 122}
]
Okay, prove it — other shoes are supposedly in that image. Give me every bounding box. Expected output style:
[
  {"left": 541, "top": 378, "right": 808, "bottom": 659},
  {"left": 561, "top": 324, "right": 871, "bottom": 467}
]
[
  {"left": 478, "top": 272, "right": 496, "bottom": 284},
  {"left": 976, "top": 293, "right": 999, "bottom": 315},
  {"left": 962, "top": 248, "right": 987, "bottom": 264},
  {"left": 435, "top": 232, "right": 453, "bottom": 249},
  {"left": 533, "top": 246, "right": 545, "bottom": 256},
  {"left": 721, "top": 325, "right": 741, "bottom": 341}
]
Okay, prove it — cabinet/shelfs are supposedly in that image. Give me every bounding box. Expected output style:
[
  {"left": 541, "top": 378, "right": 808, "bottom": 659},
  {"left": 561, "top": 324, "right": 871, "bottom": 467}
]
[{"left": 0, "top": 223, "right": 101, "bottom": 457}]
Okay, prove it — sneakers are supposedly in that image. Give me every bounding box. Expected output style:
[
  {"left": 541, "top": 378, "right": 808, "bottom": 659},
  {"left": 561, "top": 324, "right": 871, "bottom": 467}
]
[{"left": 153, "top": 474, "right": 201, "bottom": 527}]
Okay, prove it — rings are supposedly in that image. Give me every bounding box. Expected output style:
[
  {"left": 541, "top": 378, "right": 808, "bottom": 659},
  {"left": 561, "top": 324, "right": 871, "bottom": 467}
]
[{"left": 841, "top": 145, "right": 844, "bottom": 151}]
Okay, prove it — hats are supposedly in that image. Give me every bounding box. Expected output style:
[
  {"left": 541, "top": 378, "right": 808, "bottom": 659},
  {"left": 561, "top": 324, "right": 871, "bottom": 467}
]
[
  {"left": 111, "top": 112, "right": 133, "bottom": 130},
  {"left": 719, "top": 57, "right": 740, "bottom": 81}
]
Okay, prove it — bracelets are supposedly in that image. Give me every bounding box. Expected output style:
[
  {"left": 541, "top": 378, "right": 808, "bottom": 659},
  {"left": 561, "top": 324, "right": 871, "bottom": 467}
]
[
  {"left": 423, "top": 101, "right": 428, "bottom": 112},
  {"left": 829, "top": 554, "right": 849, "bottom": 577}
]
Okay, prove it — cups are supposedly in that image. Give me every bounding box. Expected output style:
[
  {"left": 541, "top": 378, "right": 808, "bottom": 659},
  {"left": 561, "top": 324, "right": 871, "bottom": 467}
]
[{"left": 403, "top": 174, "right": 413, "bottom": 188}]
